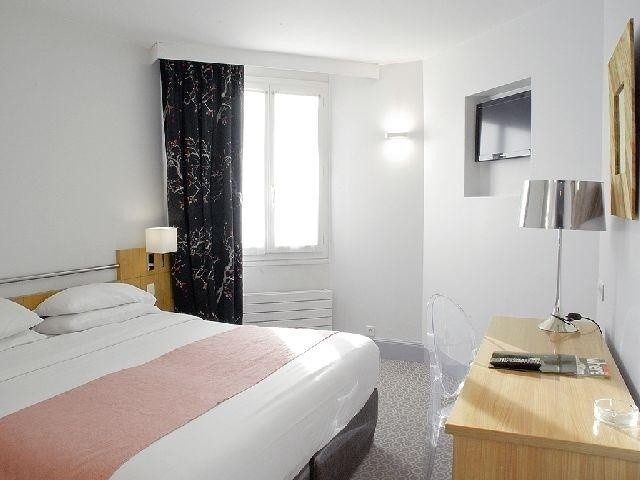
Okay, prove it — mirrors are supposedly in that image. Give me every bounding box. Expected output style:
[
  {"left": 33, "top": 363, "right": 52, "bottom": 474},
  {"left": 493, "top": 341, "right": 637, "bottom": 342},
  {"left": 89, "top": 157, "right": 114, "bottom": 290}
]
[{"left": 603, "top": 14, "right": 639, "bottom": 221}]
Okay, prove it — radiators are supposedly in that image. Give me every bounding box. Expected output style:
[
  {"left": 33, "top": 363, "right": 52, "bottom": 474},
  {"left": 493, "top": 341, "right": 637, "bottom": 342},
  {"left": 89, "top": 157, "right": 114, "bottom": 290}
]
[{"left": 241, "top": 288, "right": 334, "bottom": 333}]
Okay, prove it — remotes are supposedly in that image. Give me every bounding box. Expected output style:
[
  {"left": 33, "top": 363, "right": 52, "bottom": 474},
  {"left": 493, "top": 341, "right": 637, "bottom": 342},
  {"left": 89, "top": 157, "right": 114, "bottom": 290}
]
[{"left": 489, "top": 357, "right": 541, "bottom": 370}]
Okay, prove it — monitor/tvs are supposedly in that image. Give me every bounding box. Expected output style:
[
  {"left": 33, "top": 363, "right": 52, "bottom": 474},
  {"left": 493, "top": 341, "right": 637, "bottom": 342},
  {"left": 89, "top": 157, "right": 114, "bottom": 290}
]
[{"left": 475, "top": 90, "right": 532, "bottom": 162}]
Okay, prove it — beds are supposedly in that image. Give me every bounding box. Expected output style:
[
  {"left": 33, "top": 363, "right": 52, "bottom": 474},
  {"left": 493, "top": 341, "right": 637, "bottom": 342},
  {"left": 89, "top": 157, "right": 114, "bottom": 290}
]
[{"left": 0, "top": 265, "right": 383, "bottom": 480}]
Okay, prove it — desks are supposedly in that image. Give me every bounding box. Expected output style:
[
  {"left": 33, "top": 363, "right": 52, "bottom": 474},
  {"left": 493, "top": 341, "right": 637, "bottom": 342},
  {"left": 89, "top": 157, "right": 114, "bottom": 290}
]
[{"left": 443, "top": 312, "right": 640, "bottom": 480}]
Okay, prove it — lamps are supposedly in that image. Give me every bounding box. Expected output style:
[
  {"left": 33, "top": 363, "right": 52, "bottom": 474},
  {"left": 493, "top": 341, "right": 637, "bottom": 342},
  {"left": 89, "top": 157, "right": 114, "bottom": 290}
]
[
  {"left": 519, "top": 178, "right": 608, "bottom": 336},
  {"left": 144, "top": 226, "right": 179, "bottom": 275}
]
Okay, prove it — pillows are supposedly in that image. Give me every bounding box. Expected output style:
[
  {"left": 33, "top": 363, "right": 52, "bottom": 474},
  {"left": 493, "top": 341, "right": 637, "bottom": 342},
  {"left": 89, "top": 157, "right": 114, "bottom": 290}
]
[{"left": 0, "top": 281, "right": 158, "bottom": 351}]
